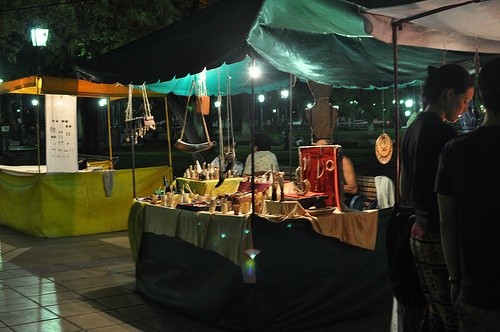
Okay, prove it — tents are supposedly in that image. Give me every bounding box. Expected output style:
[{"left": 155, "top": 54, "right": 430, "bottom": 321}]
[
  {"left": 247, "top": 0, "right": 500, "bottom": 332},
  {"left": 0, "top": 76, "right": 173, "bottom": 238}
]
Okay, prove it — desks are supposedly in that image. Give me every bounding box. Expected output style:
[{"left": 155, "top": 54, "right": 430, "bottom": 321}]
[{"left": 128, "top": 187, "right": 397, "bottom": 332}]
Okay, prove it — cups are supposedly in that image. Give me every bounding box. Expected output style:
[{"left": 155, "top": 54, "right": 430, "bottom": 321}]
[{"left": 151, "top": 193, "right": 250, "bottom": 215}]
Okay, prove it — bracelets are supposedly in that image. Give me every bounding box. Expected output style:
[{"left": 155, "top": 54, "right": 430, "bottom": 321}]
[{"left": 448, "top": 277, "right": 460, "bottom": 284}]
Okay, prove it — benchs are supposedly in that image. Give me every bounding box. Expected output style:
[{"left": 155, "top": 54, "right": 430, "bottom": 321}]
[{"left": 278, "top": 166, "right": 395, "bottom": 210}]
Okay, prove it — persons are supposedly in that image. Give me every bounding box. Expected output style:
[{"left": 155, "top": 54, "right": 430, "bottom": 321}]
[
  {"left": 297, "top": 139, "right": 357, "bottom": 194},
  {"left": 210, "top": 137, "right": 243, "bottom": 175},
  {"left": 243, "top": 134, "right": 279, "bottom": 175},
  {"left": 399, "top": 64, "right": 475, "bottom": 332},
  {"left": 434, "top": 58, "right": 500, "bottom": 332}
]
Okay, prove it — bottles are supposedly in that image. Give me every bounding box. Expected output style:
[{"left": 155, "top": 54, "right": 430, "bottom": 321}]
[{"left": 163, "top": 175, "right": 172, "bottom": 207}]
